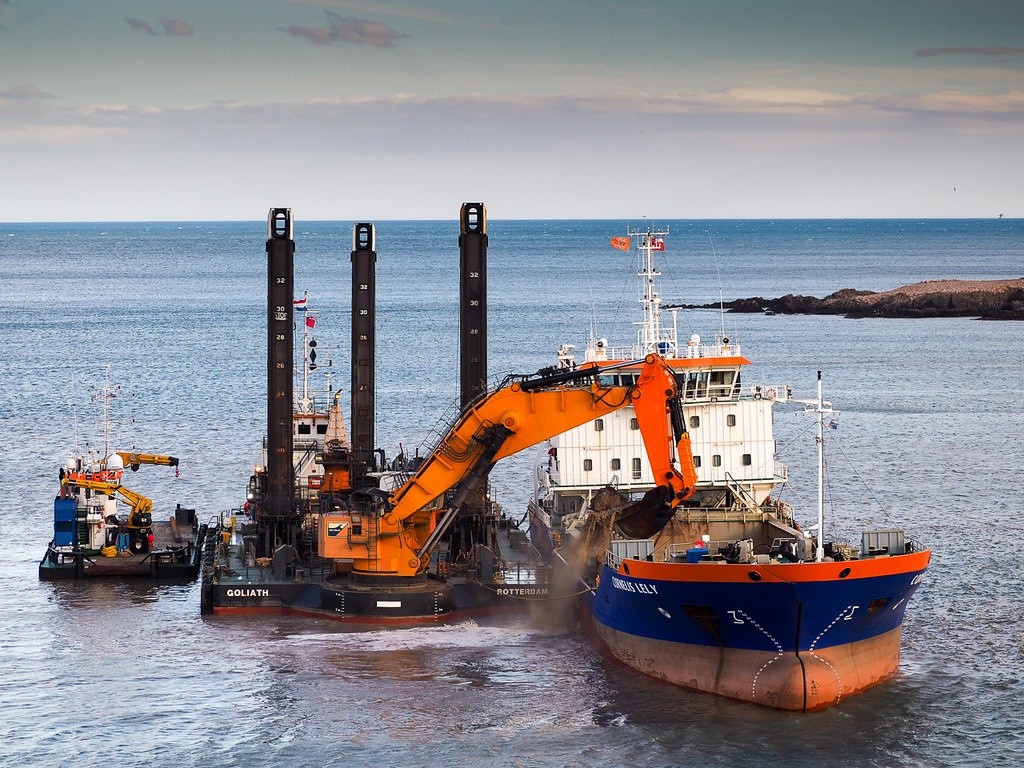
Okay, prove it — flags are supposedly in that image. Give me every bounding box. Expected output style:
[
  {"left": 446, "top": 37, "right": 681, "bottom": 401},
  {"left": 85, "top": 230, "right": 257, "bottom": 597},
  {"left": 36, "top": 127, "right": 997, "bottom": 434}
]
[
  {"left": 610, "top": 237, "right": 630, "bottom": 251},
  {"left": 650, "top": 237, "right": 664, "bottom": 251},
  {"left": 293, "top": 299, "right": 307, "bottom": 312}
]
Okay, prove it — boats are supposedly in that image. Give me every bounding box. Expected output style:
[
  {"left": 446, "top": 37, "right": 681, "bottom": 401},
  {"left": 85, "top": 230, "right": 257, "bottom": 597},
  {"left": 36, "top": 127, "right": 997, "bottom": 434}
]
[
  {"left": 200, "top": 201, "right": 570, "bottom": 628},
  {"left": 37, "top": 365, "right": 207, "bottom": 580},
  {"left": 261, "top": 289, "right": 349, "bottom": 498},
  {"left": 525, "top": 221, "right": 932, "bottom": 715}
]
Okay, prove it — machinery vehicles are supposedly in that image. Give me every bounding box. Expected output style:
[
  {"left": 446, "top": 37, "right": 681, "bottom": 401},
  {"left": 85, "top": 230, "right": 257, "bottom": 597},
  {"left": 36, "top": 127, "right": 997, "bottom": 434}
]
[{"left": 316, "top": 343, "right": 752, "bottom": 589}]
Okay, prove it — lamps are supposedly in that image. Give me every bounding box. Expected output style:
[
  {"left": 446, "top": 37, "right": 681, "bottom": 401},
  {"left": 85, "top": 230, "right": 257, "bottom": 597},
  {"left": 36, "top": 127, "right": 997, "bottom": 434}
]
[
  {"left": 721, "top": 336, "right": 729, "bottom": 346},
  {"left": 597, "top": 338, "right": 607, "bottom": 348},
  {"left": 711, "top": 397, "right": 718, "bottom": 402},
  {"left": 755, "top": 393, "right": 761, "bottom": 400},
  {"left": 691, "top": 334, "right": 701, "bottom": 343}
]
